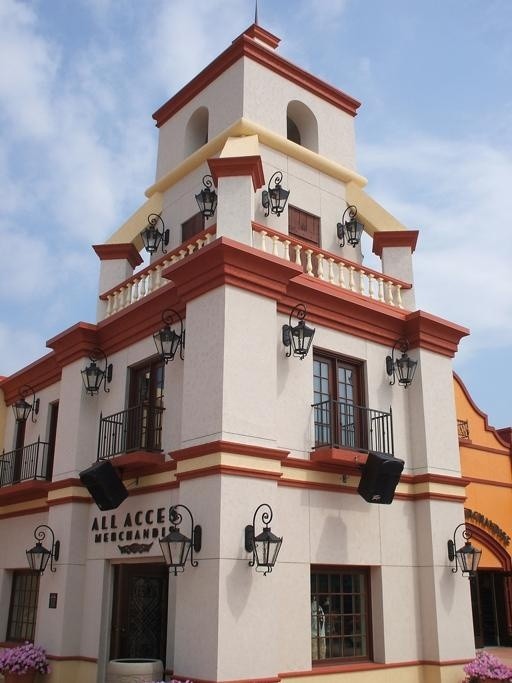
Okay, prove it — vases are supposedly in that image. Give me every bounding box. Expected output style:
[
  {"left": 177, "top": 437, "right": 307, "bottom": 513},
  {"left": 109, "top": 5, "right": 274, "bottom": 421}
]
[
  {"left": 4, "top": 666, "right": 35, "bottom": 683},
  {"left": 478, "top": 678, "right": 502, "bottom": 683}
]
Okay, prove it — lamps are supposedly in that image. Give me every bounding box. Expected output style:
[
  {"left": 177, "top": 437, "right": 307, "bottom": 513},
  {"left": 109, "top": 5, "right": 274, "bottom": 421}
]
[
  {"left": 12, "top": 384, "right": 40, "bottom": 423},
  {"left": 447, "top": 523, "right": 483, "bottom": 581},
  {"left": 245, "top": 505, "right": 284, "bottom": 577},
  {"left": 153, "top": 308, "right": 185, "bottom": 364},
  {"left": 158, "top": 504, "right": 202, "bottom": 576},
  {"left": 262, "top": 171, "right": 290, "bottom": 217},
  {"left": 141, "top": 213, "right": 170, "bottom": 254},
  {"left": 386, "top": 337, "right": 417, "bottom": 389},
  {"left": 81, "top": 347, "right": 112, "bottom": 396},
  {"left": 283, "top": 304, "right": 316, "bottom": 359},
  {"left": 26, "top": 524, "right": 61, "bottom": 575},
  {"left": 195, "top": 175, "right": 217, "bottom": 220},
  {"left": 337, "top": 205, "right": 364, "bottom": 247}
]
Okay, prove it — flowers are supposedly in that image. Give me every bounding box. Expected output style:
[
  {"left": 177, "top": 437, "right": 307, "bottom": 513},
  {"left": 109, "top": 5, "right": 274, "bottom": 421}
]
[
  {"left": 463, "top": 651, "right": 512, "bottom": 681},
  {"left": 0, "top": 641, "right": 54, "bottom": 676}
]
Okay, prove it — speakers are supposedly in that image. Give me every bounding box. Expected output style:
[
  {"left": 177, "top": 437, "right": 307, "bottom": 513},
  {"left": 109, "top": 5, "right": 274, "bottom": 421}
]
[
  {"left": 78, "top": 459, "right": 129, "bottom": 511},
  {"left": 357, "top": 449, "right": 404, "bottom": 505}
]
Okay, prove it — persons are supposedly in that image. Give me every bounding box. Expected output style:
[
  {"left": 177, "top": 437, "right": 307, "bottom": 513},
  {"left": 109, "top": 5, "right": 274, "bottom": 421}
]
[{"left": 311, "top": 599, "right": 326, "bottom": 659}]
